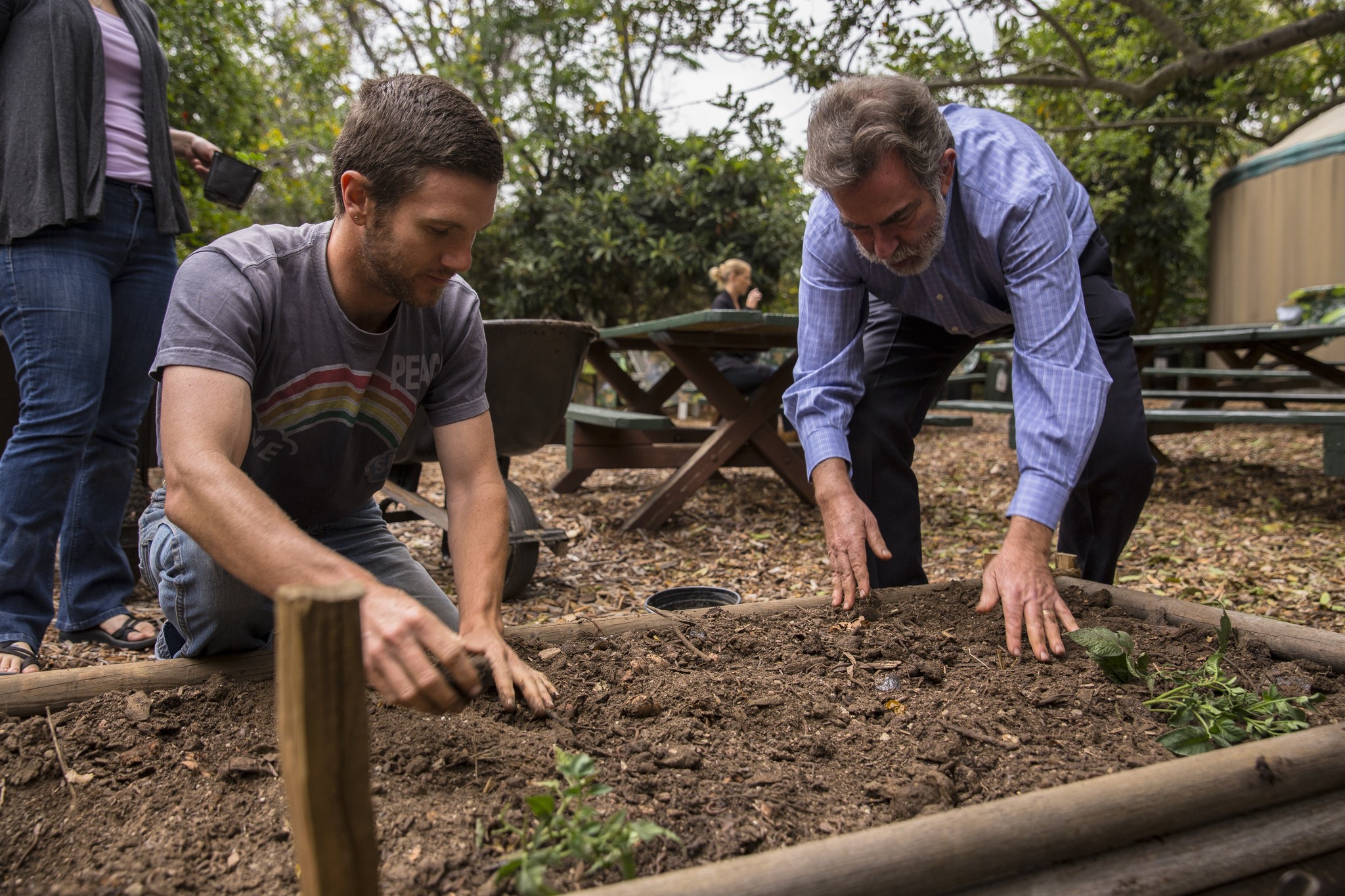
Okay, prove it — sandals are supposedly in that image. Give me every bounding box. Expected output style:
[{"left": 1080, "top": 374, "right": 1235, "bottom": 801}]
[
  {"left": 59, "top": 614, "right": 160, "bottom": 648},
  {"left": 0, "top": 640, "right": 40, "bottom": 676}
]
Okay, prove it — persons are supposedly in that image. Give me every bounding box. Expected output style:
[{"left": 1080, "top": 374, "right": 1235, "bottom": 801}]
[
  {"left": 705, "top": 259, "right": 804, "bottom": 445},
  {"left": 0, "top": 0, "right": 225, "bottom": 677},
  {"left": 781, "top": 79, "right": 1157, "bottom": 666},
  {"left": 140, "top": 75, "right": 561, "bottom": 722}
]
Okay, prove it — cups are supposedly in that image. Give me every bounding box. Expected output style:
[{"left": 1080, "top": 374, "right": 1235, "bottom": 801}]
[{"left": 204, "top": 151, "right": 263, "bottom": 211}]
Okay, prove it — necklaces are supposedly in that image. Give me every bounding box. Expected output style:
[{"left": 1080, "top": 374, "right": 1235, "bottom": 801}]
[{"left": 93, "top": 0, "right": 103, "bottom": 10}]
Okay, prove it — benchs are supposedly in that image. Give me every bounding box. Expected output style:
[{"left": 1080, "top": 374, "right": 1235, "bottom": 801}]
[
  {"left": 550, "top": 399, "right": 673, "bottom": 495},
  {"left": 1143, "top": 410, "right": 1344, "bottom": 423},
  {"left": 1139, "top": 365, "right": 1312, "bottom": 381}
]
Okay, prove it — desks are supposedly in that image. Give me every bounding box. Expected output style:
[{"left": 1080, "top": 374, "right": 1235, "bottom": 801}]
[
  {"left": 584, "top": 307, "right": 818, "bottom": 539},
  {"left": 974, "top": 322, "right": 1345, "bottom": 475},
  {"left": 1141, "top": 388, "right": 1345, "bottom": 401}
]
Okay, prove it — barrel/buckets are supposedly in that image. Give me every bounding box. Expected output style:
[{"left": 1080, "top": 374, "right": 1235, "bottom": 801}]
[{"left": 646, "top": 587, "right": 742, "bottom": 614}]
